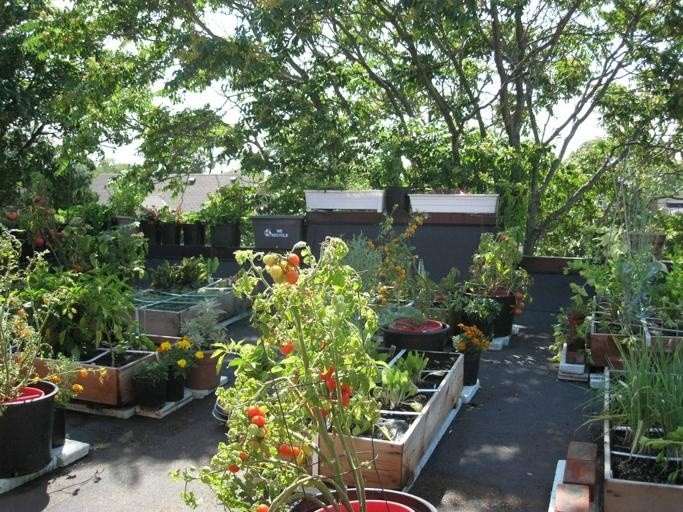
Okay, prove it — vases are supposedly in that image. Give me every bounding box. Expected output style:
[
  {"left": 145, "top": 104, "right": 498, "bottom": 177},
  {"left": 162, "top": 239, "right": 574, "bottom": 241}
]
[{"left": 302, "top": 188, "right": 387, "bottom": 213}]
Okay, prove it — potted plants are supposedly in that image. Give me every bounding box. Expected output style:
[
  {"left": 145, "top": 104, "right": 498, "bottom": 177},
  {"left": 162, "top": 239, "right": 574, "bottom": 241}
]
[
  {"left": 556, "top": 227, "right": 683, "bottom": 509},
  {"left": 401, "top": 130, "right": 558, "bottom": 212},
  {"left": 177, "top": 216, "right": 536, "bottom": 511},
  {"left": 0, "top": 191, "right": 176, "bottom": 477}
]
